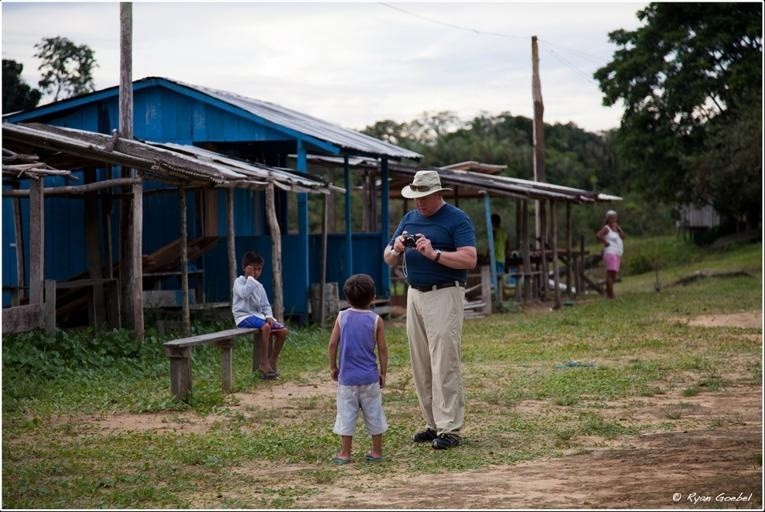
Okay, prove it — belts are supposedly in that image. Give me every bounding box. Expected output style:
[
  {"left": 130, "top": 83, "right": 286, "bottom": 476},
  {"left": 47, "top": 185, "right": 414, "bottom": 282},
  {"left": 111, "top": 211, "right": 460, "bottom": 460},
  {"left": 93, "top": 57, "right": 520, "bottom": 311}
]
[{"left": 412, "top": 281, "right": 465, "bottom": 292}]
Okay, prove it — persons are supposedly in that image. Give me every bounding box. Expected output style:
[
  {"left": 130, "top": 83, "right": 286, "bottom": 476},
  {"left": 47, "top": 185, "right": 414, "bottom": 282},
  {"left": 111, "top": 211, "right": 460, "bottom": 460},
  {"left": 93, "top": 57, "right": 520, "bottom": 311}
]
[
  {"left": 232, "top": 251, "right": 288, "bottom": 378},
  {"left": 328, "top": 274, "right": 388, "bottom": 464},
  {"left": 486, "top": 214, "right": 511, "bottom": 301},
  {"left": 596, "top": 209, "right": 625, "bottom": 298},
  {"left": 384, "top": 170, "right": 478, "bottom": 450}
]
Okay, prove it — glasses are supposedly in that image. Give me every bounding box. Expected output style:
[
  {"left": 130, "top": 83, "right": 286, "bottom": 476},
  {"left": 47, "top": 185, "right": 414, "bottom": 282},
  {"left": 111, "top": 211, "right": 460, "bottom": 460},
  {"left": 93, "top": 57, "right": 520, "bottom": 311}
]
[{"left": 410, "top": 183, "right": 441, "bottom": 192}]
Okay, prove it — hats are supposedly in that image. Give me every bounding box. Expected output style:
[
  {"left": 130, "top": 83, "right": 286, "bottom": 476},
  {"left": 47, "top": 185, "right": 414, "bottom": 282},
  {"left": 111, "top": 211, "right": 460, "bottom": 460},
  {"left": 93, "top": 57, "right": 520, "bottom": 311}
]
[{"left": 401, "top": 171, "right": 453, "bottom": 199}]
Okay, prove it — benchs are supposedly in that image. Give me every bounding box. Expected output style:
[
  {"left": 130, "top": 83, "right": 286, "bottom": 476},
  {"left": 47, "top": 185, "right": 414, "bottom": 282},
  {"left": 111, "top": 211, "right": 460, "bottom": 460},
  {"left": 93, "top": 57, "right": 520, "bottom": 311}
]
[{"left": 162, "top": 324, "right": 278, "bottom": 402}]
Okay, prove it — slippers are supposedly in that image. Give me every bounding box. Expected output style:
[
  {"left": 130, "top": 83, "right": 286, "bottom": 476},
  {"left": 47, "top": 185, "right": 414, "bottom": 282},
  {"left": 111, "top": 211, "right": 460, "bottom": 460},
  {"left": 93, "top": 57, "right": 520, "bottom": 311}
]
[
  {"left": 334, "top": 454, "right": 350, "bottom": 465},
  {"left": 365, "top": 450, "right": 383, "bottom": 462}
]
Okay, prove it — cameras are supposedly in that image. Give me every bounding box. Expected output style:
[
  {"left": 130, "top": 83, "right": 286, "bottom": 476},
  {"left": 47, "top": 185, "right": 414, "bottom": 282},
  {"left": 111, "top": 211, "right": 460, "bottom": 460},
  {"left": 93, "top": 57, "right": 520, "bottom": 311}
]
[{"left": 402, "top": 234, "right": 420, "bottom": 247}]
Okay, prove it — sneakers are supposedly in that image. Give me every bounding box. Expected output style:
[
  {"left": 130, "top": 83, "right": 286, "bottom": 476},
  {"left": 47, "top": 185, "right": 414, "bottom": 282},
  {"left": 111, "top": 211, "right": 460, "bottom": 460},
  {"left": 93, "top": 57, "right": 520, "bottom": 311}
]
[{"left": 412, "top": 429, "right": 459, "bottom": 449}]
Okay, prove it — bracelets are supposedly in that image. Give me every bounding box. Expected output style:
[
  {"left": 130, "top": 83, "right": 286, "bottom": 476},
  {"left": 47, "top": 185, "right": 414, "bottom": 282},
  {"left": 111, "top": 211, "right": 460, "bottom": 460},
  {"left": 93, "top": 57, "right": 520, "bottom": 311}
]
[
  {"left": 434, "top": 249, "right": 441, "bottom": 262},
  {"left": 391, "top": 247, "right": 404, "bottom": 256}
]
